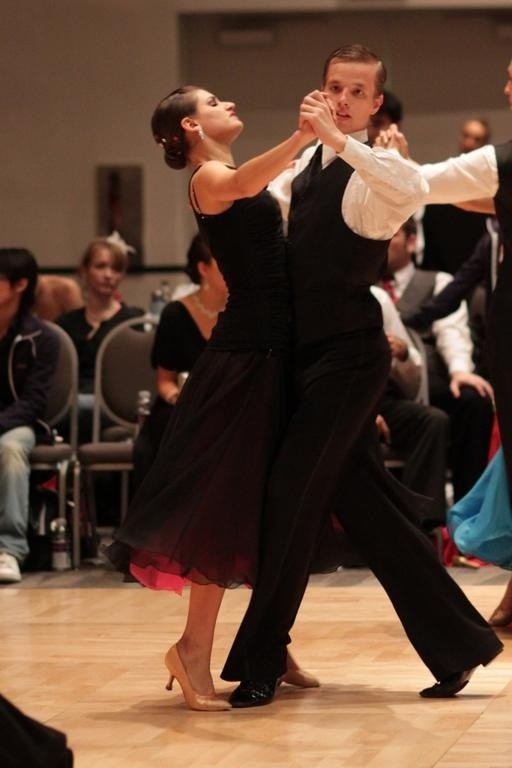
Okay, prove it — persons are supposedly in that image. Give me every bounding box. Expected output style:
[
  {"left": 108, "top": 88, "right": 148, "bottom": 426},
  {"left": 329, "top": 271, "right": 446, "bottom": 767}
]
[
  {"left": 117, "top": 86, "right": 337, "bottom": 710},
  {"left": 377, "top": 124, "right": 512, "bottom": 626},
  {"left": 375, "top": 57, "right": 511, "bottom": 525},
  {"left": 0, "top": 692, "right": 75, "bottom": 767},
  {"left": 223, "top": 44, "right": 502, "bottom": 707},
  {"left": 0, "top": 230, "right": 232, "bottom": 581},
  {"left": 370, "top": 90, "right": 502, "bottom": 569}
]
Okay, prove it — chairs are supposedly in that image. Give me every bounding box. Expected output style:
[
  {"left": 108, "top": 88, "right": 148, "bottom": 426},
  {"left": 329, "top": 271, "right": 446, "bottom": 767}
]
[
  {"left": 9, "top": 319, "right": 77, "bottom": 567},
  {"left": 72, "top": 314, "right": 162, "bottom": 571}
]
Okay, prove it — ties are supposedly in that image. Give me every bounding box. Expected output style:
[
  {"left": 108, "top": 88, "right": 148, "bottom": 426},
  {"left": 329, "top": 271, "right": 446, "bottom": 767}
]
[
  {"left": 302, "top": 144, "right": 328, "bottom": 188},
  {"left": 379, "top": 279, "right": 399, "bottom": 303}
]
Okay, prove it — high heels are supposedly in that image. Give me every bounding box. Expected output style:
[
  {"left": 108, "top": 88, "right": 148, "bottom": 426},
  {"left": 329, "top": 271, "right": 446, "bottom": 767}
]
[
  {"left": 164, "top": 644, "right": 231, "bottom": 711},
  {"left": 278, "top": 666, "right": 318, "bottom": 688}
]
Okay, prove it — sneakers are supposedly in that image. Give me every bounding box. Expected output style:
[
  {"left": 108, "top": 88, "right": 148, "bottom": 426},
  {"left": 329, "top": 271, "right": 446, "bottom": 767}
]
[{"left": 0, "top": 552, "right": 21, "bottom": 581}]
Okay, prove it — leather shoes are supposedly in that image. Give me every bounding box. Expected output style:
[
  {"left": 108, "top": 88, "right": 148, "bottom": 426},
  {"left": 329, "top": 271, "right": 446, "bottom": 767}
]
[
  {"left": 228, "top": 666, "right": 286, "bottom": 707},
  {"left": 420, "top": 640, "right": 503, "bottom": 697}
]
